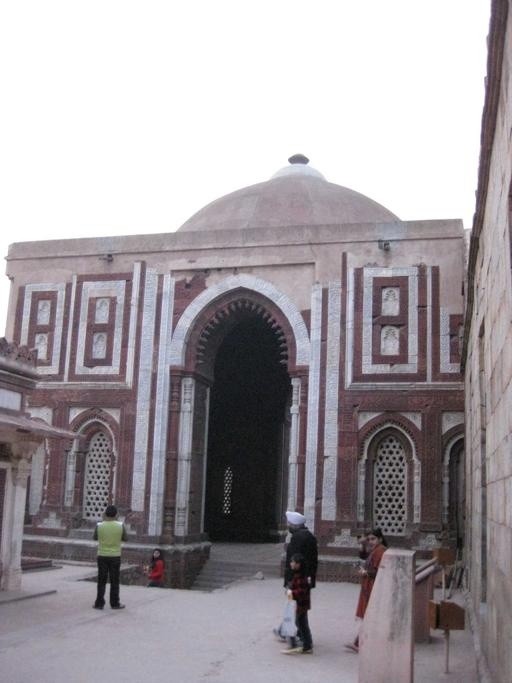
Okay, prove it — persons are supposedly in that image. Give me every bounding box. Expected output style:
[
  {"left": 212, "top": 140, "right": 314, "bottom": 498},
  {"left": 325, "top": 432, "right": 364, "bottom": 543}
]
[
  {"left": 144, "top": 548, "right": 164, "bottom": 587},
  {"left": 92, "top": 505, "right": 128, "bottom": 609},
  {"left": 273, "top": 511, "right": 319, "bottom": 643},
  {"left": 344, "top": 528, "right": 389, "bottom": 652},
  {"left": 281, "top": 553, "right": 314, "bottom": 655}
]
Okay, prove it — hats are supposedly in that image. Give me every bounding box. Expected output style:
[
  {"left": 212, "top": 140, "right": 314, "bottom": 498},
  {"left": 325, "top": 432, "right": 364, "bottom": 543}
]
[{"left": 285, "top": 511, "right": 307, "bottom": 525}]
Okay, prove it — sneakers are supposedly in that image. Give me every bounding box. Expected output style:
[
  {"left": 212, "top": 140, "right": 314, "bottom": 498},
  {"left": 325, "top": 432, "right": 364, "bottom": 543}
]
[
  {"left": 281, "top": 647, "right": 313, "bottom": 655},
  {"left": 344, "top": 644, "right": 359, "bottom": 653},
  {"left": 112, "top": 602, "right": 126, "bottom": 609},
  {"left": 92, "top": 603, "right": 104, "bottom": 610}
]
